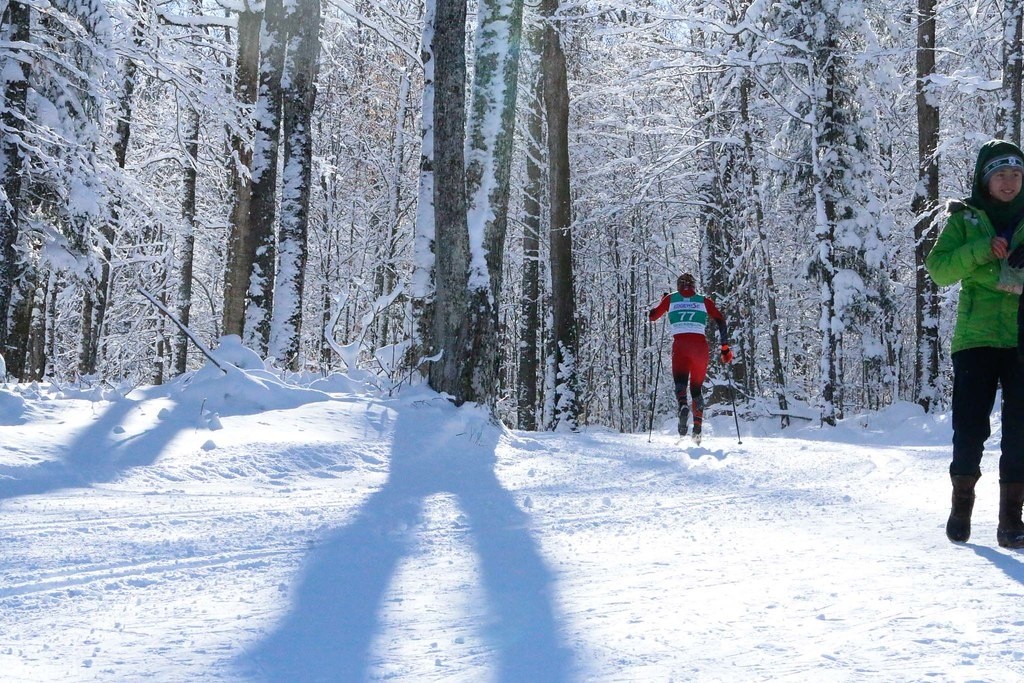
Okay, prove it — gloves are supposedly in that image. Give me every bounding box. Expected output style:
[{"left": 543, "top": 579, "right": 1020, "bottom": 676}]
[
  {"left": 1007, "top": 244, "right": 1024, "bottom": 269},
  {"left": 721, "top": 343, "right": 733, "bottom": 364}
]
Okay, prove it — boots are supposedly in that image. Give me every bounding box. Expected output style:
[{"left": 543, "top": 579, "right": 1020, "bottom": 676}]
[
  {"left": 947, "top": 473, "right": 983, "bottom": 544},
  {"left": 997, "top": 483, "right": 1024, "bottom": 548}
]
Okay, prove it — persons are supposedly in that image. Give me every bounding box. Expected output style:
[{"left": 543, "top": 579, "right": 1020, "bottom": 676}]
[
  {"left": 926, "top": 140, "right": 1024, "bottom": 548},
  {"left": 649, "top": 273, "right": 733, "bottom": 443}
]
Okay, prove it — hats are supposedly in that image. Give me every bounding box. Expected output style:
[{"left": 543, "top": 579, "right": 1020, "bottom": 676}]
[
  {"left": 980, "top": 148, "right": 1024, "bottom": 184},
  {"left": 678, "top": 273, "right": 696, "bottom": 288}
]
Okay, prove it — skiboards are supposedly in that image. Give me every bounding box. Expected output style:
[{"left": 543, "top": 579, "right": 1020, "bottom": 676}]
[{"left": 674, "top": 435, "right": 703, "bottom": 447}]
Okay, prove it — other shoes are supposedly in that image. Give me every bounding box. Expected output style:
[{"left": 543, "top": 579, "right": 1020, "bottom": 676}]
[
  {"left": 693, "top": 426, "right": 702, "bottom": 434},
  {"left": 678, "top": 404, "right": 690, "bottom": 436}
]
